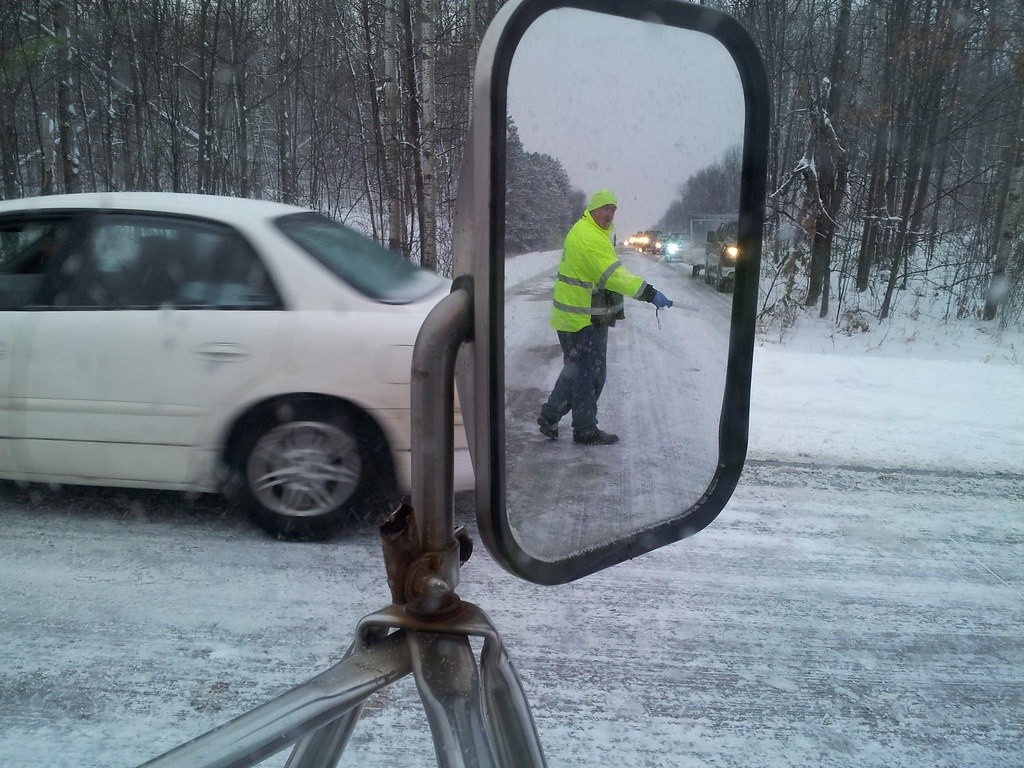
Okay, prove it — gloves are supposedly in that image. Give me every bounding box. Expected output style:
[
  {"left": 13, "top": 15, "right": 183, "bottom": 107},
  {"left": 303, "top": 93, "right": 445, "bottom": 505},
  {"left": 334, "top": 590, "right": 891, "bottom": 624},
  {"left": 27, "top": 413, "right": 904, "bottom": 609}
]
[{"left": 652, "top": 291, "right": 670, "bottom": 309}]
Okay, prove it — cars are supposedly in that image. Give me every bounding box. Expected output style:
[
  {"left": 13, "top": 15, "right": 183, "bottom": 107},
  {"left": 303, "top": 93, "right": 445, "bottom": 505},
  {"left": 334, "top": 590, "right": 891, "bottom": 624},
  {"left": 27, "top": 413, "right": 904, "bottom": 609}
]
[
  {"left": 625, "top": 217, "right": 717, "bottom": 278},
  {"left": 0, "top": 192, "right": 476, "bottom": 540}
]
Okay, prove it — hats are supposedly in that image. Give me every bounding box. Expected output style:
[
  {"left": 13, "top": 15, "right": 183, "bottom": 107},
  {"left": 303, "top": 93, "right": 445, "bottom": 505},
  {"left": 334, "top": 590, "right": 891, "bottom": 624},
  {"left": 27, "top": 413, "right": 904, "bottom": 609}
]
[{"left": 588, "top": 189, "right": 617, "bottom": 212}]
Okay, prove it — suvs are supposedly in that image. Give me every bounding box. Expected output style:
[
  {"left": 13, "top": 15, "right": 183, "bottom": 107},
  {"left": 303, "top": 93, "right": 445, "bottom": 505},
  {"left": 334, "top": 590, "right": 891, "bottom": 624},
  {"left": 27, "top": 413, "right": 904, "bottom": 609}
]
[{"left": 704, "top": 220, "right": 738, "bottom": 294}]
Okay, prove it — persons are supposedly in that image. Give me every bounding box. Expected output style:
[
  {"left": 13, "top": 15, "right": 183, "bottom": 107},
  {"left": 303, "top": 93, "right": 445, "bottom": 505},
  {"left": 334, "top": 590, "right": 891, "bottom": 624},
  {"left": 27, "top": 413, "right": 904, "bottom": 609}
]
[
  {"left": 614, "top": 234, "right": 617, "bottom": 246},
  {"left": 537, "top": 189, "right": 668, "bottom": 446}
]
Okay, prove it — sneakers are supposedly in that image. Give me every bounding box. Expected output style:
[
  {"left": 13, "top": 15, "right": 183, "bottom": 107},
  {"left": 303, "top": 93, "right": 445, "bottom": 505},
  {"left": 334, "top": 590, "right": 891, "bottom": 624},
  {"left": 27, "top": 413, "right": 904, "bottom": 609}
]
[
  {"left": 573, "top": 429, "right": 619, "bottom": 445},
  {"left": 537, "top": 414, "right": 560, "bottom": 441}
]
